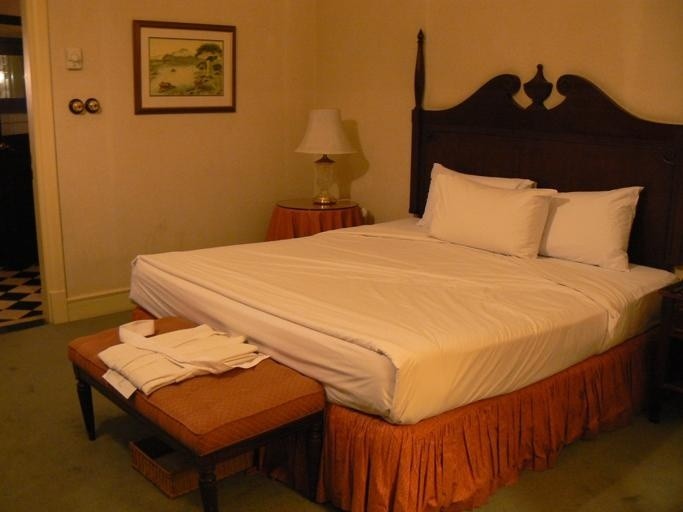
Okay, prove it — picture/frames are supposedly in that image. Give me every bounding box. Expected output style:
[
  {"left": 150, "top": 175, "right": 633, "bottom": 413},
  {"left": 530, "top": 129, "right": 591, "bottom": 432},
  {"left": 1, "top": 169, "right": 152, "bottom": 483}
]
[{"left": 132, "top": 19, "right": 236, "bottom": 116}]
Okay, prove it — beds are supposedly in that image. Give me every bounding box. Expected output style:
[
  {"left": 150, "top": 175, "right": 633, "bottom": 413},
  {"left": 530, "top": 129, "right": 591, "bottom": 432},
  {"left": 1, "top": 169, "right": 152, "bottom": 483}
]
[{"left": 129, "top": 30, "right": 683, "bottom": 512}]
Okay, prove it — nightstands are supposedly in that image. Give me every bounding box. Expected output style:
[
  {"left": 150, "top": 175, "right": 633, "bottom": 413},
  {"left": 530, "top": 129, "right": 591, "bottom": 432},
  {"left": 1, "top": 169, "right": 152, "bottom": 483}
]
[{"left": 646, "top": 280, "right": 683, "bottom": 423}]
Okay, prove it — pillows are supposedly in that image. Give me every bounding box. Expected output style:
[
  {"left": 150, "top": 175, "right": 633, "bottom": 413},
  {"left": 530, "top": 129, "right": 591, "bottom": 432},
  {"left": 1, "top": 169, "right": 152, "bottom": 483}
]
[
  {"left": 538, "top": 186, "right": 644, "bottom": 273},
  {"left": 427, "top": 169, "right": 557, "bottom": 263},
  {"left": 416, "top": 162, "right": 537, "bottom": 227}
]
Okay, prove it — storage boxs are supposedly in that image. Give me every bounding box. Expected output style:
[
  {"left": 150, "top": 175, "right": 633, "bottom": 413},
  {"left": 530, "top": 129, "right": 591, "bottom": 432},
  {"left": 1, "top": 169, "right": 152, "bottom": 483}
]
[{"left": 129, "top": 434, "right": 254, "bottom": 498}]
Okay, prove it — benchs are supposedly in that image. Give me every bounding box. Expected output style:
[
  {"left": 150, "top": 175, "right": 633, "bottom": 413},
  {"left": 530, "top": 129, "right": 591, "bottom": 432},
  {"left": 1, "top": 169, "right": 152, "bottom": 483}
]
[{"left": 69, "top": 317, "right": 327, "bottom": 512}]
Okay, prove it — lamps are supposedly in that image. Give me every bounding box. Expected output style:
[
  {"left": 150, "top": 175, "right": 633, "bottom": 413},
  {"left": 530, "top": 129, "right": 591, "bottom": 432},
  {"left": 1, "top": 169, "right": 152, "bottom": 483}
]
[{"left": 294, "top": 108, "right": 358, "bottom": 204}]
